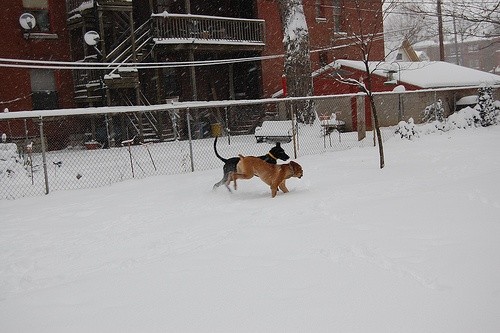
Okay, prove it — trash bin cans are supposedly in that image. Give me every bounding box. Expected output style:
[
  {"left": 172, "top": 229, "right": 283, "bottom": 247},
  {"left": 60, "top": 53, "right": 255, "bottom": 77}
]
[{"left": 211, "top": 122, "right": 223, "bottom": 137}]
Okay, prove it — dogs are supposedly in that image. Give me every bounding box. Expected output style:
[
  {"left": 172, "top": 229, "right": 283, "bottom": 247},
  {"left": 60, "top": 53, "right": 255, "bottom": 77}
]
[
  {"left": 226, "top": 154, "right": 303, "bottom": 198},
  {"left": 213, "top": 135, "right": 290, "bottom": 193}
]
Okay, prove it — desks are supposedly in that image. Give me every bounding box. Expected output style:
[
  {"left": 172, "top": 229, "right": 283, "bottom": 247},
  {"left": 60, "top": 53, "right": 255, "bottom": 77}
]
[{"left": 321, "top": 120, "right": 345, "bottom": 148}]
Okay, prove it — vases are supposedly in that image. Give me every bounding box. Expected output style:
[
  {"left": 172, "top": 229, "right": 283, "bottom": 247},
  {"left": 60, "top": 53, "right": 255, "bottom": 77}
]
[{"left": 84, "top": 144, "right": 98, "bottom": 150}]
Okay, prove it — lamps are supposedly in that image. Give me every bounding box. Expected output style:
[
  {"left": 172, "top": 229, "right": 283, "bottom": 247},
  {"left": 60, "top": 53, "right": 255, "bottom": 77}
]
[
  {"left": 85, "top": 31, "right": 107, "bottom": 58},
  {"left": 19, "top": 13, "right": 36, "bottom": 42}
]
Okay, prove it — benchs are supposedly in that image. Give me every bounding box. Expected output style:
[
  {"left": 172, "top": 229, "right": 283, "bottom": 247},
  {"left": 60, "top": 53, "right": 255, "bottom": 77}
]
[{"left": 255, "top": 120, "right": 297, "bottom": 144}]
